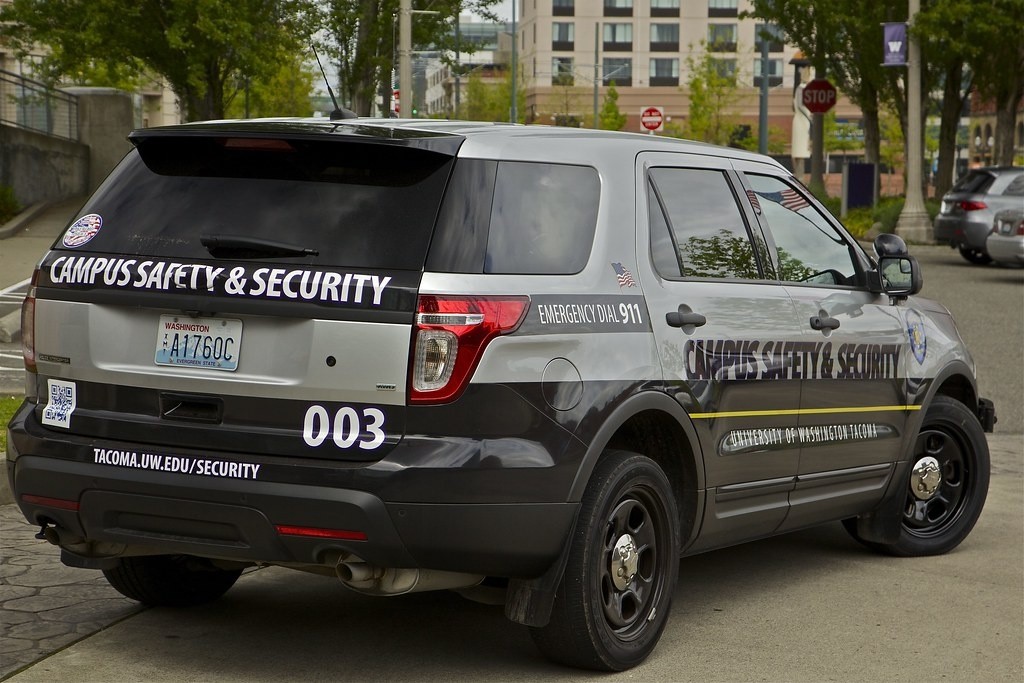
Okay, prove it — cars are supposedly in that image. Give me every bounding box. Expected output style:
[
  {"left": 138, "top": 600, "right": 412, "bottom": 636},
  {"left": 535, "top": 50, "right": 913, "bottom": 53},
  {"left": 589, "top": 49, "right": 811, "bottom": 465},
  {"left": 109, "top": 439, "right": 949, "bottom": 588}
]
[{"left": 985, "top": 205, "right": 1024, "bottom": 268}]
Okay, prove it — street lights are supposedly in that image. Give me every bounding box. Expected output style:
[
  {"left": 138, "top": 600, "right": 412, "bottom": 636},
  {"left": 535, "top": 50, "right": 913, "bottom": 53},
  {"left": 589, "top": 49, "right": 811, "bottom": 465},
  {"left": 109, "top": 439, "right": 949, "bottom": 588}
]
[
  {"left": 757, "top": 17, "right": 790, "bottom": 155},
  {"left": 973, "top": 135, "right": 995, "bottom": 164}
]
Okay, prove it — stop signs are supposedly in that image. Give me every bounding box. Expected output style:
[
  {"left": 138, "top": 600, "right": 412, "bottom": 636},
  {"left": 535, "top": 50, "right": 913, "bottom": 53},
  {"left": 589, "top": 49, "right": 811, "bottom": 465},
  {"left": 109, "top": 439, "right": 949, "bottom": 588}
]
[
  {"left": 801, "top": 78, "right": 838, "bottom": 113},
  {"left": 640, "top": 107, "right": 664, "bottom": 132}
]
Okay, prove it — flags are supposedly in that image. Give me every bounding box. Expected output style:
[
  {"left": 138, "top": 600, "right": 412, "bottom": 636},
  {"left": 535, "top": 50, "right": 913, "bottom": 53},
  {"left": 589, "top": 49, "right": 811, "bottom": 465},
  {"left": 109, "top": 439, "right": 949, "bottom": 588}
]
[{"left": 755, "top": 187, "right": 810, "bottom": 213}]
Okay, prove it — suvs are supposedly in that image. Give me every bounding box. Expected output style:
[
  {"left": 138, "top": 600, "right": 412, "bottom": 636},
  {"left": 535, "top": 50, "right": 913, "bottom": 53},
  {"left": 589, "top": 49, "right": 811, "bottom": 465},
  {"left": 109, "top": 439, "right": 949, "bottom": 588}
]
[
  {"left": 932, "top": 164, "right": 1024, "bottom": 262},
  {"left": 1, "top": 106, "right": 997, "bottom": 674}
]
